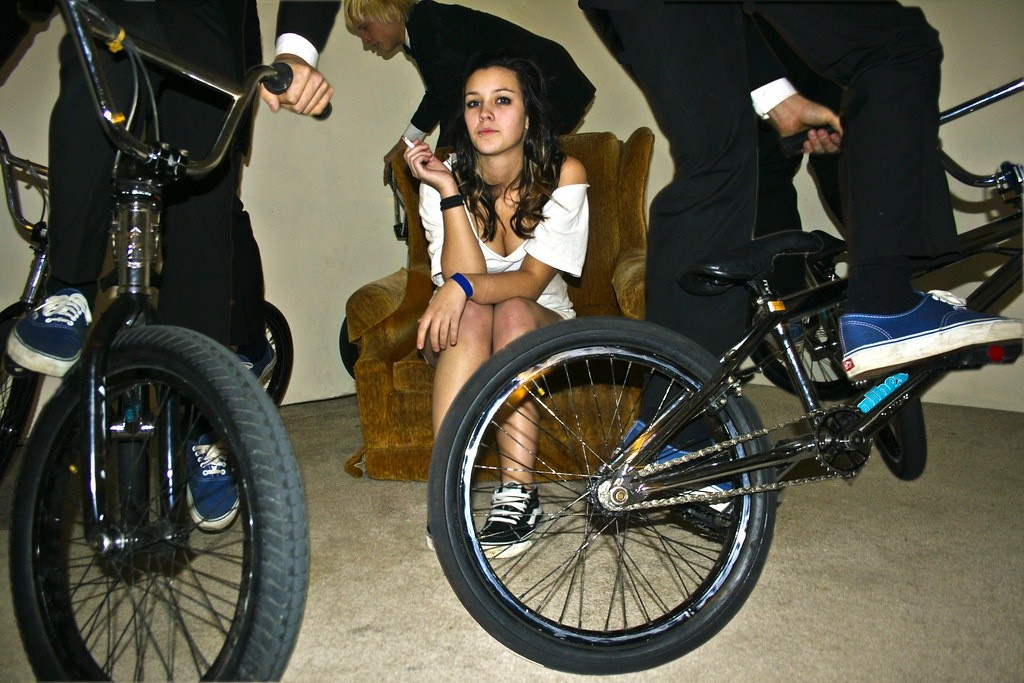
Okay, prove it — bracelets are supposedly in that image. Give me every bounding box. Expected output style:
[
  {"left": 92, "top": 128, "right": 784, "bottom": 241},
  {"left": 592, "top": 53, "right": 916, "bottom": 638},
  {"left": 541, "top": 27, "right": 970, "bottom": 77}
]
[
  {"left": 439, "top": 194, "right": 465, "bottom": 211},
  {"left": 451, "top": 271, "right": 474, "bottom": 299}
]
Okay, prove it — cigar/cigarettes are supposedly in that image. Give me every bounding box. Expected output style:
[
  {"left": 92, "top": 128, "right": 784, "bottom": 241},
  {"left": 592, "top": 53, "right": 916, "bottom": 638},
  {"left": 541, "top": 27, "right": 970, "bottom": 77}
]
[{"left": 401, "top": 135, "right": 416, "bottom": 149}]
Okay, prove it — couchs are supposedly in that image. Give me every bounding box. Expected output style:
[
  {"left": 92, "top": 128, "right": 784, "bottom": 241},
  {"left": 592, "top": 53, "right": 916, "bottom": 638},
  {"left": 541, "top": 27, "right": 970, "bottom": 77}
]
[{"left": 346, "top": 126, "right": 655, "bottom": 482}]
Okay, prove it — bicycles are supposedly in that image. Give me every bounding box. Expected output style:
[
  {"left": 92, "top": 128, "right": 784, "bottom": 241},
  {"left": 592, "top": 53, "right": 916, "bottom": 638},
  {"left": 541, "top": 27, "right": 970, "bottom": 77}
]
[
  {"left": 0, "top": 2, "right": 334, "bottom": 683},
  {"left": 425, "top": 77, "right": 1024, "bottom": 676}
]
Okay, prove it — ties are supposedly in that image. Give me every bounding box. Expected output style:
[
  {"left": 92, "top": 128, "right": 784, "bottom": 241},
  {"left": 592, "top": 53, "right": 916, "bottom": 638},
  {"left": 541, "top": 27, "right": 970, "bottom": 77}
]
[{"left": 402, "top": 44, "right": 414, "bottom": 56}]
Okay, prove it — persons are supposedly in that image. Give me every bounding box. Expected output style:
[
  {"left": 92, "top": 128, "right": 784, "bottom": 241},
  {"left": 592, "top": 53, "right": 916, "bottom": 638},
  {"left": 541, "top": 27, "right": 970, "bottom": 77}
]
[
  {"left": 344, "top": 0, "right": 596, "bottom": 167},
  {"left": 0, "top": 0, "right": 341, "bottom": 534},
  {"left": 578, "top": 0, "right": 1024, "bottom": 546},
  {"left": 402, "top": 56, "right": 590, "bottom": 558}
]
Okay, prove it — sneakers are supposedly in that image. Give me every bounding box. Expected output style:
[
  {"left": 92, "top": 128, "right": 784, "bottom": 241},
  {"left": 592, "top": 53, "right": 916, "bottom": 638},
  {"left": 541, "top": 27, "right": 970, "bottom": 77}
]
[
  {"left": 186, "top": 432, "right": 240, "bottom": 531},
  {"left": 426, "top": 523, "right": 436, "bottom": 553},
  {"left": 836, "top": 286, "right": 1024, "bottom": 382},
  {"left": 475, "top": 480, "right": 543, "bottom": 559},
  {"left": 7, "top": 287, "right": 95, "bottom": 377},
  {"left": 609, "top": 420, "right": 740, "bottom": 516}
]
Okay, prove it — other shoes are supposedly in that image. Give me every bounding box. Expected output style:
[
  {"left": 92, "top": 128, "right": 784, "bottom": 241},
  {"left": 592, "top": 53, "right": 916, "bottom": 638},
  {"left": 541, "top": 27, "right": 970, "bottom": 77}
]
[{"left": 235, "top": 343, "right": 279, "bottom": 382}]
